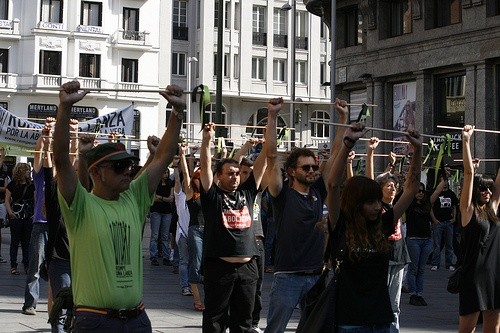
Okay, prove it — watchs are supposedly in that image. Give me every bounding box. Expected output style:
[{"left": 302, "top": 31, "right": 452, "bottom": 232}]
[{"left": 170, "top": 105, "right": 183, "bottom": 120}]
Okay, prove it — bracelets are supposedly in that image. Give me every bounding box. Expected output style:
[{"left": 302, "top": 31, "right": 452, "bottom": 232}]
[
  {"left": 344, "top": 136, "right": 357, "bottom": 149},
  {"left": 388, "top": 161, "right": 395, "bottom": 168}
]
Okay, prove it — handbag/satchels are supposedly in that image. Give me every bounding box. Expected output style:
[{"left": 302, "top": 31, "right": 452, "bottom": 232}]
[{"left": 293, "top": 227, "right": 346, "bottom": 333}]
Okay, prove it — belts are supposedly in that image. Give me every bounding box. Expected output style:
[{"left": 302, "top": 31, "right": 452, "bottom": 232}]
[
  {"left": 107, "top": 309, "right": 142, "bottom": 320},
  {"left": 289, "top": 271, "right": 322, "bottom": 276}
]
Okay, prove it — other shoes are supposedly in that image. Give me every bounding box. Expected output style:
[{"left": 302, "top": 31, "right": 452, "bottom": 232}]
[
  {"left": 181, "top": 287, "right": 192, "bottom": 296},
  {"left": 151, "top": 260, "right": 160, "bottom": 266},
  {"left": 409, "top": 295, "right": 428, "bottom": 306},
  {"left": 10, "top": 268, "right": 16, "bottom": 274},
  {"left": 192, "top": 302, "right": 205, "bottom": 311},
  {"left": 225, "top": 328, "right": 230, "bottom": 333},
  {"left": 22, "top": 307, "right": 37, "bottom": 315},
  {"left": 0, "top": 257, "right": 7, "bottom": 263},
  {"left": 173, "top": 268, "right": 179, "bottom": 274},
  {"left": 449, "top": 266, "right": 455, "bottom": 271},
  {"left": 250, "top": 327, "right": 263, "bottom": 333},
  {"left": 163, "top": 260, "right": 172, "bottom": 266},
  {"left": 431, "top": 266, "right": 437, "bottom": 271}
]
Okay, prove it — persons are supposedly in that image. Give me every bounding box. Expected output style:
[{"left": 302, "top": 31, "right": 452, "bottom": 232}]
[{"left": 0, "top": 80, "right": 500, "bottom": 333}]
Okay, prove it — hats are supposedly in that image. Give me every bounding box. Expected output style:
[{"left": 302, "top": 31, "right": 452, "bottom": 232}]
[{"left": 85, "top": 142, "right": 140, "bottom": 173}]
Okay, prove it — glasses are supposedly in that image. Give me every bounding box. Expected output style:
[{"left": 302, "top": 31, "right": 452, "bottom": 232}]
[
  {"left": 100, "top": 162, "right": 138, "bottom": 175},
  {"left": 479, "top": 184, "right": 496, "bottom": 192},
  {"left": 295, "top": 164, "right": 320, "bottom": 172},
  {"left": 418, "top": 189, "right": 425, "bottom": 193},
  {"left": 228, "top": 172, "right": 239, "bottom": 176}
]
